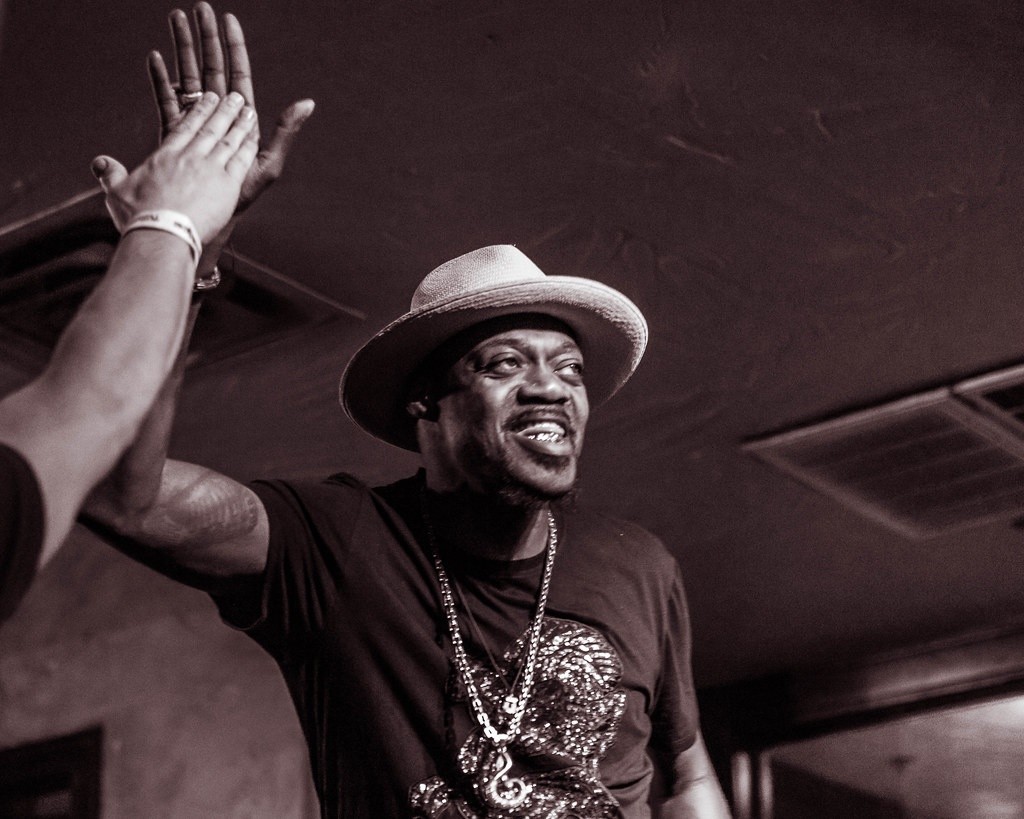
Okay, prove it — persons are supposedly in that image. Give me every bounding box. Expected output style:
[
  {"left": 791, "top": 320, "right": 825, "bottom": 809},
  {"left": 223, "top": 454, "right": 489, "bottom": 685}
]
[
  {"left": 75, "top": 1, "right": 734, "bottom": 819},
  {"left": 0, "top": 87, "right": 261, "bottom": 623}
]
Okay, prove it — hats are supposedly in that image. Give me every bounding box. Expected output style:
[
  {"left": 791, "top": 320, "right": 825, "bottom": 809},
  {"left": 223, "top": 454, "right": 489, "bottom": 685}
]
[{"left": 339, "top": 244, "right": 650, "bottom": 454}]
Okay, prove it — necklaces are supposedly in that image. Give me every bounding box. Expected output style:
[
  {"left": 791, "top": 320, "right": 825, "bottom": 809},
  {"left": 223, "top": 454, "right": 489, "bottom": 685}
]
[{"left": 432, "top": 508, "right": 559, "bottom": 746}]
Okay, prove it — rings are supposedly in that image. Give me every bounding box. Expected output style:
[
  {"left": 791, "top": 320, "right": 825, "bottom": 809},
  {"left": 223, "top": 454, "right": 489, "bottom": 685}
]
[{"left": 182, "top": 91, "right": 202, "bottom": 104}]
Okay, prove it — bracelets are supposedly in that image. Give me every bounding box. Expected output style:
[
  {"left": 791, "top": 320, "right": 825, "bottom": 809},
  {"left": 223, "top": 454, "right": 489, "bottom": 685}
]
[
  {"left": 191, "top": 267, "right": 223, "bottom": 295},
  {"left": 120, "top": 209, "right": 204, "bottom": 268}
]
